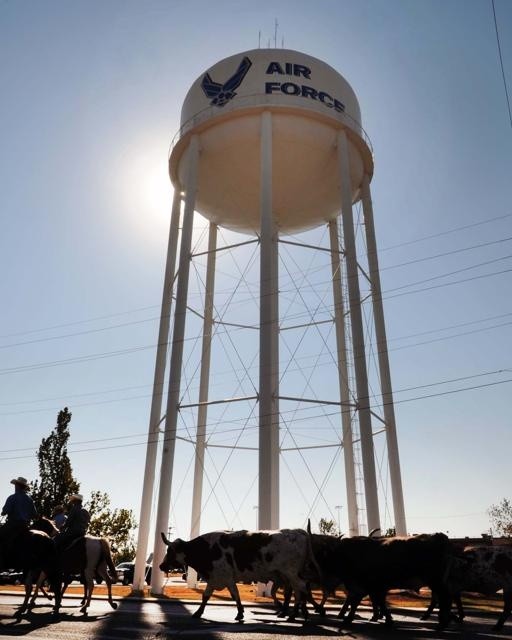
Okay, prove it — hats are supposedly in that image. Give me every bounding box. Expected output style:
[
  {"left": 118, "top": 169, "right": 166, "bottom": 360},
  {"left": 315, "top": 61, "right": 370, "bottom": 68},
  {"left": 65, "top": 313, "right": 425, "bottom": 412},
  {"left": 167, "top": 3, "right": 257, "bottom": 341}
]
[
  {"left": 66, "top": 495, "right": 82, "bottom": 503},
  {"left": 10, "top": 477, "right": 30, "bottom": 491}
]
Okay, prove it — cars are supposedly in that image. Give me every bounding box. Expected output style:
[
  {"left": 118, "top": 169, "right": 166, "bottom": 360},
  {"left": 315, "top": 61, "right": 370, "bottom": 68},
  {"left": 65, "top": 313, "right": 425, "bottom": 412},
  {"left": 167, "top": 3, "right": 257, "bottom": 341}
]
[
  {"left": 107, "top": 563, "right": 152, "bottom": 585},
  {"left": 0, "top": 570, "right": 25, "bottom": 585}
]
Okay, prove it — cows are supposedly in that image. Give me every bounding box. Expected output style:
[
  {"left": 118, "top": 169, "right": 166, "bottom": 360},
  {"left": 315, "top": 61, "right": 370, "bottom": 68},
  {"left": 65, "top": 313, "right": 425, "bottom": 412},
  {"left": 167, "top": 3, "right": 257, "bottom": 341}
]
[{"left": 156, "top": 516, "right": 512, "bottom": 633}]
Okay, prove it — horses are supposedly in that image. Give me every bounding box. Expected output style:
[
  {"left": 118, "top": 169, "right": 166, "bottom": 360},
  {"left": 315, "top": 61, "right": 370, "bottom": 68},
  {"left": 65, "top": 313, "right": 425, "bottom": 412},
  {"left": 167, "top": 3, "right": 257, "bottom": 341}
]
[{"left": 0, "top": 514, "right": 119, "bottom": 614}]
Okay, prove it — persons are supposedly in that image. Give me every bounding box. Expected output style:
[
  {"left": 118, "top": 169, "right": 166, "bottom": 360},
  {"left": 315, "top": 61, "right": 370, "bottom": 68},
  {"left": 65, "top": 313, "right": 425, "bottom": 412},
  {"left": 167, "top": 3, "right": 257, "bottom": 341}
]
[
  {"left": 51, "top": 495, "right": 90, "bottom": 592},
  {"left": 0, "top": 477, "right": 36, "bottom": 566}
]
